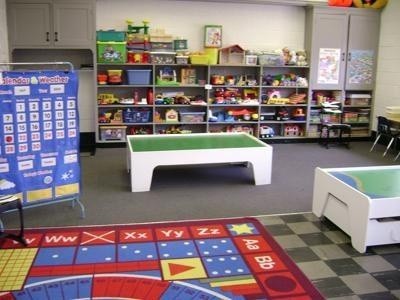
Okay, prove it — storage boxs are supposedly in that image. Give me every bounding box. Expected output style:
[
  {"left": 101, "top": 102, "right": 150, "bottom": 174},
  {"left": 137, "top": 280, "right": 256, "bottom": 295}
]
[
  {"left": 95, "top": 29, "right": 209, "bottom": 65},
  {"left": 178, "top": 110, "right": 207, "bottom": 122},
  {"left": 122, "top": 107, "right": 152, "bottom": 123},
  {"left": 100, "top": 125, "right": 128, "bottom": 143},
  {"left": 125, "top": 69, "right": 153, "bottom": 86}
]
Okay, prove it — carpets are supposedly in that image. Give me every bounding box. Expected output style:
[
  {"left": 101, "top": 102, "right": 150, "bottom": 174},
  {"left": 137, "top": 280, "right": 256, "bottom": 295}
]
[{"left": 1, "top": 209, "right": 325, "bottom": 300}]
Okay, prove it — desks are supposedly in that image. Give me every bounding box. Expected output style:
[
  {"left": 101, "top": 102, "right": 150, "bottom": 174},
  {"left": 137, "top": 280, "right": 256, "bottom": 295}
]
[
  {"left": 125, "top": 130, "right": 274, "bottom": 193},
  {"left": 311, "top": 160, "right": 399, "bottom": 254}
]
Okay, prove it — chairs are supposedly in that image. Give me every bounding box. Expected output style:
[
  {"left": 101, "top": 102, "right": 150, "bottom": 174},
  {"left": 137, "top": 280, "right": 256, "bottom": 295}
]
[
  {"left": 369, "top": 116, "right": 400, "bottom": 159},
  {"left": 318, "top": 102, "right": 352, "bottom": 150}
]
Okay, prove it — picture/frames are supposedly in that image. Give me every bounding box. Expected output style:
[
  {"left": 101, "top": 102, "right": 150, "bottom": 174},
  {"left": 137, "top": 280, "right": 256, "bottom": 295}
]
[{"left": 204, "top": 25, "right": 223, "bottom": 48}]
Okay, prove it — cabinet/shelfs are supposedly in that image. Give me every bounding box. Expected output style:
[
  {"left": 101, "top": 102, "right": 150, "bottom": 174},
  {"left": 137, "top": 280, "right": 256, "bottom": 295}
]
[
  {"left": 304, "top": 5, "right": 382, "bottom": 141},
  {"left": 96, "top": 61, "right": 310, "bottom": 144},
  {"left": 4, "top": 0, "right": 97, "bottom": 53}
]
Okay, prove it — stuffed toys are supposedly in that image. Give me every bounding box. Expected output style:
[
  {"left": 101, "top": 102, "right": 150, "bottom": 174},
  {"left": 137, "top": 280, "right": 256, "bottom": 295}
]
[{"left": 283, "top": 47, "right": 308, "bottom": 66}]
